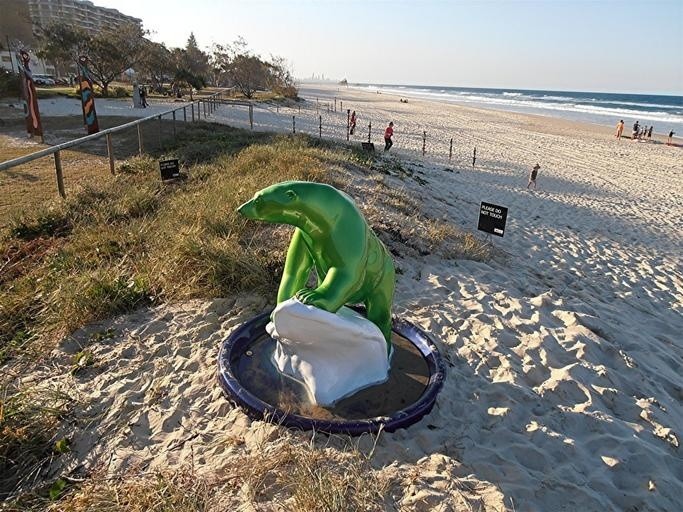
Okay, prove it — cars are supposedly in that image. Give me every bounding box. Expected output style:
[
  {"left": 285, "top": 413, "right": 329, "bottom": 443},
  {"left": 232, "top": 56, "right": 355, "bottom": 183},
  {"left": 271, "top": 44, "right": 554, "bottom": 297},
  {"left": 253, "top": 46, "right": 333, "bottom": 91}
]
[{"left": 30, "top": 72, "right": 77, "bottom": 87}]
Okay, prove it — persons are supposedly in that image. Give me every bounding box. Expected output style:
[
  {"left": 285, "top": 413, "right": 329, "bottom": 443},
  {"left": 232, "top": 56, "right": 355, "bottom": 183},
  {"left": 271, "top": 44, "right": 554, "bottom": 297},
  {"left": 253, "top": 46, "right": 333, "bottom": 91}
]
[
  {"left": 666, "top": 128, "right": 676, "bottom": 146},
  {"left": 630, "top": 120, "right": 653, "bottom": 142},
  {"left": 350, "top": 111, "right": 356, "bottom": 129},
  {"left": 615, "top": 119, "right": 623, "bottom": 140},
  {"left": 138, "top": 85, "right": 149, "bottom": 108},
  {"left": 618, "top": 122, "right": 625, "bottom": 136},
  {"left": 525, "top": 163, "right": 540, "bottom": 189},
  {"left": 382, "top": 122, "right": 394, "bottom": 152}
]
